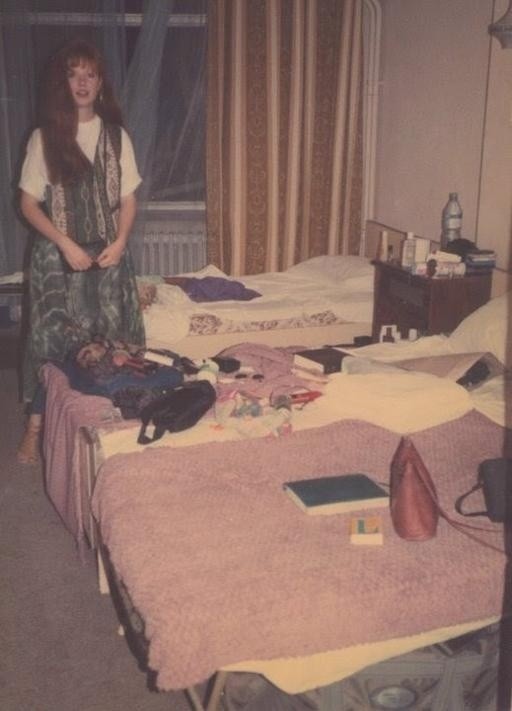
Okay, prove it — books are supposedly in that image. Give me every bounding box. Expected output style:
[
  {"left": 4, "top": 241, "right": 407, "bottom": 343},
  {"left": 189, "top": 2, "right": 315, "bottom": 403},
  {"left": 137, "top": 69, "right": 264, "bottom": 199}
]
[
  {"left": 280, "top": 472, "right": 392, "bottom": 519},
  {"left": 291, "top": 349, "right": 353, "bottom": 378},
  {"left": 144, "top": 349, "right": 200, "bottom": 375}
]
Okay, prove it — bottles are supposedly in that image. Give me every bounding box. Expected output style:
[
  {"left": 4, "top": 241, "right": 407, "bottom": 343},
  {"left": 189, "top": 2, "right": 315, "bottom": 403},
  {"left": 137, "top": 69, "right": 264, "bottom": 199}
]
[
  {"left": 441, "top": 193, "right": 463, "bottom": 250},
  {"left": 378, "top": 230, "right": 388, "bottom": 263}
]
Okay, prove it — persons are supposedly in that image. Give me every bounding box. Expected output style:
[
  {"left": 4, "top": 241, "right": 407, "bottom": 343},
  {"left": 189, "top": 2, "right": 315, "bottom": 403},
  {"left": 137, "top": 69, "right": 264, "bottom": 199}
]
[{"left": 16, "top": 38, "right": 146, "bottom": 469}]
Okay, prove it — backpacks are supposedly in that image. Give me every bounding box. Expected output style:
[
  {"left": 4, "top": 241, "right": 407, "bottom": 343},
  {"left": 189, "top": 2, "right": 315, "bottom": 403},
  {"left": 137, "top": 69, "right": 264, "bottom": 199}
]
[{"left": 44, "top": 334, "right": 187, "bottom": 420}]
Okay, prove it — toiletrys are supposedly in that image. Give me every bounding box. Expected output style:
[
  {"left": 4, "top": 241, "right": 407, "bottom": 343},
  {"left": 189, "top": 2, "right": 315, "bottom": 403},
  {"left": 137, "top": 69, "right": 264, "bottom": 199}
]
[{"left": 378, "top": 231, "right": 390, "bottom": 263}]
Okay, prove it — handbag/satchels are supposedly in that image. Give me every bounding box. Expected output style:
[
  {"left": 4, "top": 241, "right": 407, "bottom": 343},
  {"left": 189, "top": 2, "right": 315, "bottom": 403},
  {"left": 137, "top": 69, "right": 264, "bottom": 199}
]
[
  {"left": 455, "top": 457, "right": 511, "bottom": 522},
  {"left": 137, "top": 380, "right": 216, "bottom": 444}
]
